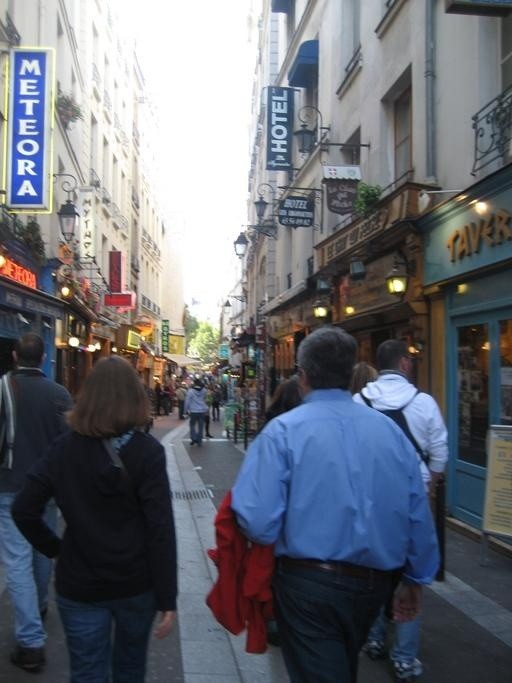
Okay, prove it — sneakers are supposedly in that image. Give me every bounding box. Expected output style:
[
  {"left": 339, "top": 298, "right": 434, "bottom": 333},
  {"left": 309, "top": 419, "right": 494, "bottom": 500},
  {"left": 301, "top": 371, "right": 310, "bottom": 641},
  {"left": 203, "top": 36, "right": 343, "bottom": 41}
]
[
  {"left": 394, "top": 658, "right": 426, "bottom": 680},
  {"left": 9, "top": 644, "right": 46, "bottom": 669},
  {"left": 363, "top": 639, "right": 386, "bottom": 657}
]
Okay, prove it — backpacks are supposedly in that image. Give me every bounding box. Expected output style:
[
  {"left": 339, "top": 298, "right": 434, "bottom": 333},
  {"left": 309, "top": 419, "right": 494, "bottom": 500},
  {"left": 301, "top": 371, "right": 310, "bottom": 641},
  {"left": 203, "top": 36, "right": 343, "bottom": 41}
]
[{"left": 358, "top": 389, "right": 424, "bottom": 459}]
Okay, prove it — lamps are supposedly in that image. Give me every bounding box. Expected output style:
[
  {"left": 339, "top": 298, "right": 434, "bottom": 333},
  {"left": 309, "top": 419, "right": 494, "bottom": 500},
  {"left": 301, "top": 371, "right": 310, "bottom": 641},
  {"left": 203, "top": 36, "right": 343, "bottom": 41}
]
[
  {"left": 51, "top": 171, "right": 80, "bottom": 242},
  {"left": 383, "top": 247, "right": 418, "bottom": 301},
  {"left": 313, "top": 286, "right": 334, "bottom": 318},
  {"left": 417, "top": 185, "right": 465, "bottom": 213},
  {"left": 224, "top": 293, "right": 248, "bottom": 307},
  {"left": 294, "top": 103, "right": 372, "bottom": 154},
  {"left": 251, "top": 181, "right": 276, "bottom": 226},
  {"left": 233, "top": 223, "right": 278, "bottom": 259}
]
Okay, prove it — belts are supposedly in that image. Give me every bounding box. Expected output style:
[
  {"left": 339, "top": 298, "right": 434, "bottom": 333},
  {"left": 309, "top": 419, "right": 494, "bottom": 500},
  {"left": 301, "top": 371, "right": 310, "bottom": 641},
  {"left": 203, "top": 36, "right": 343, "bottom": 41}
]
[{"left": 301, "top": 559, "right": 390, "bottom": 576}]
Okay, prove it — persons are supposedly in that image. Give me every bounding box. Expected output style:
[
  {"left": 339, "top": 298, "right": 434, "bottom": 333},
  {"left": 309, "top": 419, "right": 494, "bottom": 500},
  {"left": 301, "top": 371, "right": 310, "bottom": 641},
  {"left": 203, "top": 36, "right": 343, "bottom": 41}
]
[
  {"left": 230, "top": 325, "right": 448, "bottom": 683},
  {"left": 151, "top": 368, "right": 224, "bottom": 447},
  {"left": 11, "top": 354, "right": 178, "bottom": 683},
  {"left": 0, "top": 332, "right": 76, "bottom": 672}
]
